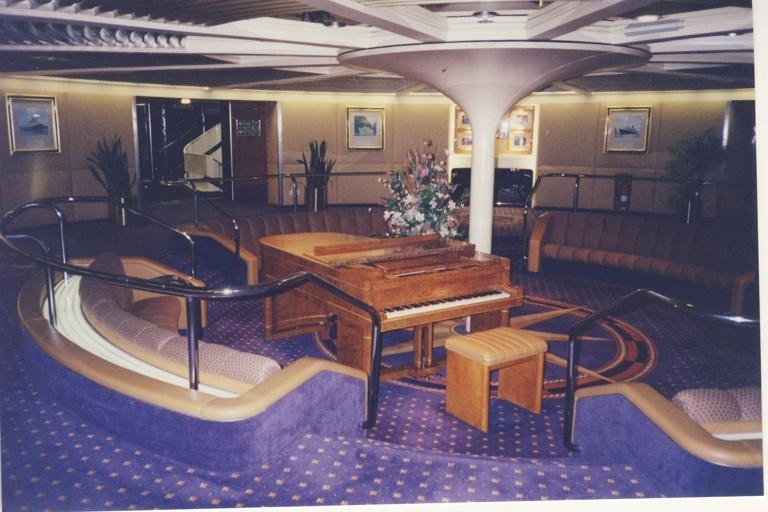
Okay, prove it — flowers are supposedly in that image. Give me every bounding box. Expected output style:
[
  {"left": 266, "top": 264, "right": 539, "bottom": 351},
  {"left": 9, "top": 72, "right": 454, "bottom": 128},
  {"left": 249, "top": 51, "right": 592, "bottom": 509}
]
[{"left": 376, "top": 138, "right": 464, "bottom": 243}]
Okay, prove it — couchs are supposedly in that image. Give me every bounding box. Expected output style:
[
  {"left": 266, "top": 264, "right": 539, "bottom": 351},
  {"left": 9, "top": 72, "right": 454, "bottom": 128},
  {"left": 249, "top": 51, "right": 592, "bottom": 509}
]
[
  {"left": 171, "top": 205, "right": 386, "bottom": 289},
  {"left": 528, "top": 208, "right": 758, "bottom": 316},
  {"left": 15, "top": 255, "right": 369, "bottom": 476}
]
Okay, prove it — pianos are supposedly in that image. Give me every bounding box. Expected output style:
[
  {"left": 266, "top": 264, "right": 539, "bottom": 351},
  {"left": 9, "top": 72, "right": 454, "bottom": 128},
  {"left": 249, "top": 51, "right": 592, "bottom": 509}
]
[{"left": 259, "top": 231, "right": 524, "bottom": 431}]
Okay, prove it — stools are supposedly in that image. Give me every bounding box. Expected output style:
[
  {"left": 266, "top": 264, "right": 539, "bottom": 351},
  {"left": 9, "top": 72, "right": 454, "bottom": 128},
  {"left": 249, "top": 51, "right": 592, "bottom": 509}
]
[{"left": 445, "top": 326, "right": 548, "bottom": 435}]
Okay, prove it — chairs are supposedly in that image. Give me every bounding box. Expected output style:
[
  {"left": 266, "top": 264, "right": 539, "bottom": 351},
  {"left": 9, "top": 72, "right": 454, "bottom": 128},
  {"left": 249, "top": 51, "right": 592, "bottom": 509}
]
[{"left": 572, "top": 367, "right": 762, "bottom": 496}]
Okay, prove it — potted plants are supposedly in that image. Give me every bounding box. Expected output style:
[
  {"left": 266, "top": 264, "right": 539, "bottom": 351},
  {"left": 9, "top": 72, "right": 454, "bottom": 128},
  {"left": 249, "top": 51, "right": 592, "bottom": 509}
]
[
  {"left": 296, "top": 137, "right": 337, "bottom": 211},
  {"left": 659, "top": 124, "right": 728, "bottom": 226},
  {"left": 88, "top": 132, "right": 137, "bottom": 226}
]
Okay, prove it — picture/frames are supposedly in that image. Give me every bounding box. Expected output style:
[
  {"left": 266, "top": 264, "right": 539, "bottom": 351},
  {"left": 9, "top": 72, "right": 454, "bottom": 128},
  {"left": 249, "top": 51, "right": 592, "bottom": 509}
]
[
  {"left": 604, "top": 107, "right": 650, "bottom": 153},
  {"left": 347, "top": 107, "right": 386, "bottom": 150},
  {"left": 4, "top": 94, "right": 61, "bottom": 155}
]
